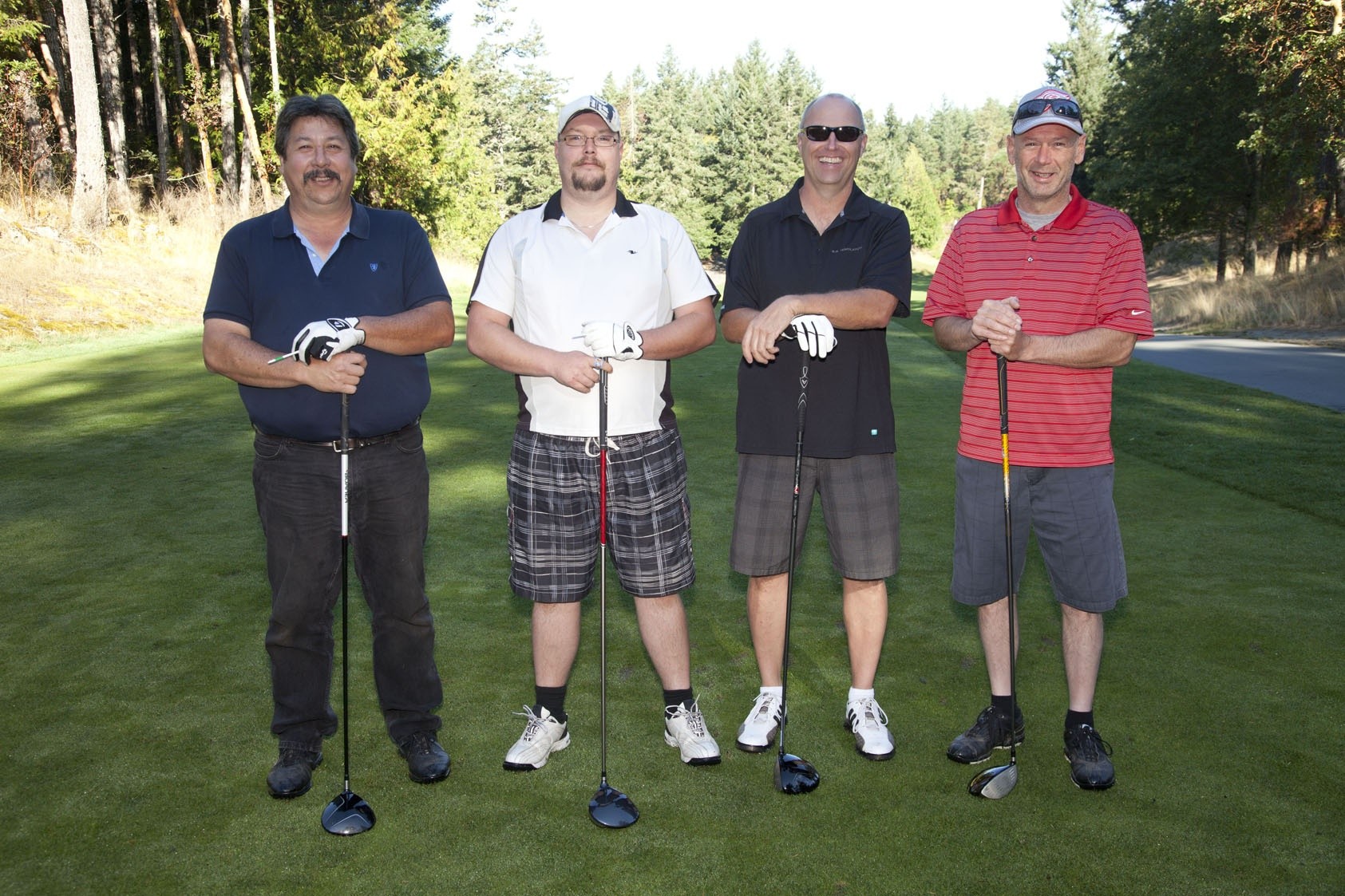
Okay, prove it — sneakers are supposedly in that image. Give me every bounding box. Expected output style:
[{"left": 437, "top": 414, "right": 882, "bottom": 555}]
[
  {"left": 1063, "top": 723, "right": 1115, "bottom": 789},
  {"left": 844, "top": 696, "right": 895, "bottom": 760},
  {"left": 664, "top": 692, "right": 722, "bottom": 767},
  {"left": 945, "top": 704, "right": 1023, "bottom": 765},
  {"left": 735, "top": 691, "right": 787, "bottom": 752},
  {"left": 503, "top": 705, "right": 570, "bottom": 771}
]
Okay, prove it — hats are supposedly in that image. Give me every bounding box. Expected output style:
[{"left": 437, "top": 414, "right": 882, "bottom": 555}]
[
  {"left": 557, "top": 94, "right": 622, "bottom": 140},
  {"left": 1011, "top": 87, "right": 1083, "bottom": 138}
]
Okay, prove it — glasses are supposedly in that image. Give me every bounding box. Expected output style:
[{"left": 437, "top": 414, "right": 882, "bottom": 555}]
[
  {"left": 558, "top": 134, "right": 618, "bottom": 147},
  {"left": 802, "top": 125, "right": 863, "bottom": 142},
  {"left": 1011, "top": 98, "right": 1084, "bottom": 129}
]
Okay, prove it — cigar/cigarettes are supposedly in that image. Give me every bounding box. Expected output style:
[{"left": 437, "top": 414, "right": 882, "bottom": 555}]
[
  {"left": 572, "top": 334, "right": 586, "bottom": 339},
  {"left": 267, "top": 350, "right": 299, "bottom": 364}
]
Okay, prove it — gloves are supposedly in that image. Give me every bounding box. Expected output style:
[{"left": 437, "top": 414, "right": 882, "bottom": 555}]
[
  {"left": 780, "top": 313, "right": 837, "bottom": 359},
  {"left": 580, "top": 318, "right": 643, "bottom": 361},
  {"left": 290, "top": 316, "right": 367, "bottom": 366}
]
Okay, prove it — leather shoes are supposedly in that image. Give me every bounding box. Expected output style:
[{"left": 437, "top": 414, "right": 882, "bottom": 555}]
[
  {"left": 266, "top": 749, "right": 324, "bottom": 798},
  {"left": 401, "top": 733, "right": 451, "bottom": 783}
]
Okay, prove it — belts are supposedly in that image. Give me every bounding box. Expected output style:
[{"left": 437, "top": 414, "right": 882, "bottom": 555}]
[{"left": 257, "top": 418, "right": 419, "bottom": 452}]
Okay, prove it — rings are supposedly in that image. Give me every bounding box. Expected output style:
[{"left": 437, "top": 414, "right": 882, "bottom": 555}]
[{"left": 593, "top": 358, "right": 603, "bottom": 369}]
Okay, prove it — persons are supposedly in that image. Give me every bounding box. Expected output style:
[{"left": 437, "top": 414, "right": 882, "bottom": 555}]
[
  {"left": 718, "top": 93, "right": 912, "bottom": 760},
  {"left": 467, "top": 96, "right": 720, "bottom": 771},
  {"left": 921, "top": 86, "right": 1152, "bottom": 794},
  {"left": 202, "top": 94, "right": 456, "bottom": 801}
]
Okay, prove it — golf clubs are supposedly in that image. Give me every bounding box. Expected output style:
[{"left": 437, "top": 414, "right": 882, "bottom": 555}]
[
  {"left": 318, "top": 391, "right": 378, "bottom": 838},
  {"left": 963, "top": 355, "right": 1024, "bottom": 804},
  {"left": 772, "top": 350, "right": 823, "bottom": 797},
  {"left": 584, "top": 357, "right": 644, "bottom": 832}
]
